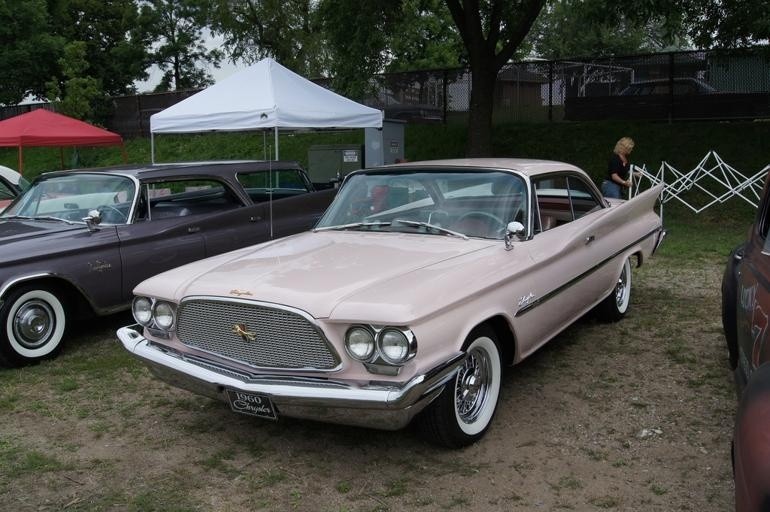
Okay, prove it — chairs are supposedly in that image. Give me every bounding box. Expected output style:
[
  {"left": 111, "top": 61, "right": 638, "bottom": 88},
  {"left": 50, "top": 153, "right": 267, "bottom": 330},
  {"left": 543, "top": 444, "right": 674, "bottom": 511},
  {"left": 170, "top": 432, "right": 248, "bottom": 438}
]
[{"left": 152, "top": 201, "right": 191, "bottom": 220}]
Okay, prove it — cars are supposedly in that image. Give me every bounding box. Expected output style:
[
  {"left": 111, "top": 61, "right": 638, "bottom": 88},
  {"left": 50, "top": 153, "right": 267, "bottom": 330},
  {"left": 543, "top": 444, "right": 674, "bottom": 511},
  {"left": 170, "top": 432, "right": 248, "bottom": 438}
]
[
  {"left": 0, "top": 156, "right": 347, "bottom": 365},
  {"left": 716, "top": 163, "right": 770, "bottom": 510},
  {"left": 617, "top": 77, "right": 722, "bottom": 96},
  {"left": 113, "top": 155, "right": 669, "bottom": 453},
  {"left": 126, "top": 108, "right": 167, "bottom": 133},
  {"left": 342, "top": 87, "right": 444, "bottom": 130}
]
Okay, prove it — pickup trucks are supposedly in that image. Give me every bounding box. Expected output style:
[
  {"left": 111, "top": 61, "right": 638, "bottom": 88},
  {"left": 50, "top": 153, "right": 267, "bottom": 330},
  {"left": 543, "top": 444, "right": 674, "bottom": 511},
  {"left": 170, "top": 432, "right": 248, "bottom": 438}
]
[{"left": 0, "top": 164, "right": 173, "bottom": 218}]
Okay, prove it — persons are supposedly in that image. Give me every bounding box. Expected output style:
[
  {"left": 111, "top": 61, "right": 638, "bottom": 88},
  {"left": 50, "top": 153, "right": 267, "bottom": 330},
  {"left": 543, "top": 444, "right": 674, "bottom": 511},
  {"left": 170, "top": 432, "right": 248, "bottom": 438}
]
[{"left": 600, "top": 137, "right": 644, "bottom": 197}]
